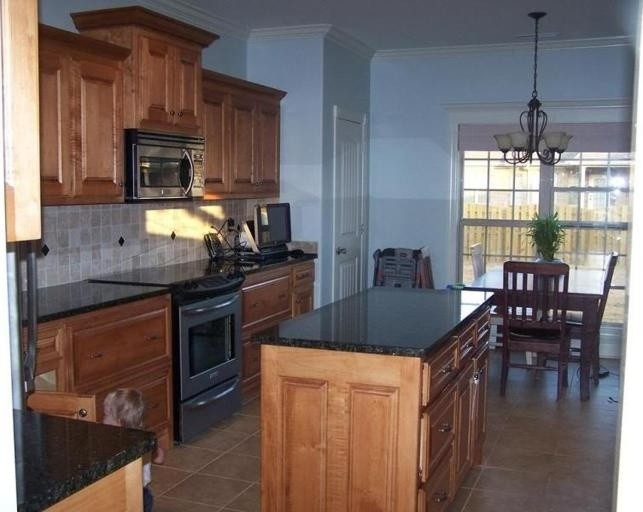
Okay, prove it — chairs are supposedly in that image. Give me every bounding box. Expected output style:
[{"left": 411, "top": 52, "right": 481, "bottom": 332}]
[
  {"left": 500, "top": 261, "right": 570, "bottom": 400},
  {"left": 471, "top": 242, "right": 504, "bottom": 350},
  {"left": 539, "top": 251, "right": 617, "bottom": 402},
  {"left": 369, "top": 248, "right": 420, "bottom": 289}
]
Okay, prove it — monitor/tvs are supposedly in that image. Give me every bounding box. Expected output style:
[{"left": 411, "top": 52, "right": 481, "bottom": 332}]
[{"left": 253, "top": 202, "right": 292, "bottom": 247}]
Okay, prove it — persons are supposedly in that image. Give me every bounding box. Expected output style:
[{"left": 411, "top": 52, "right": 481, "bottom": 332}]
[{"left": 103, "top": 388, "right": 165, "bottom": 512}]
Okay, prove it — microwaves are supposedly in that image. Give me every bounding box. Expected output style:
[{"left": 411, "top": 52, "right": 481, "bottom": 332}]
[{"left": 123, "top": 128, "right": 206, "bottom": 204}]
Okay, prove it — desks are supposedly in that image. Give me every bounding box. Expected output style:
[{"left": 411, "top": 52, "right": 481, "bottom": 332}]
[{"left": 464, "top": 267, "right": 605, "bottom": 401}]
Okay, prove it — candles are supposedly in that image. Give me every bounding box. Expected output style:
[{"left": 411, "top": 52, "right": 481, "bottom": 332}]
[{"left": 525, "top": 211, "right": 566, "bottom": 278}]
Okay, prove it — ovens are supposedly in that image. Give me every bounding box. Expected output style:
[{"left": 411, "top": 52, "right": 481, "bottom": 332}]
[{"left": 172, "top": 270, "right": 245, "bottom": 447}]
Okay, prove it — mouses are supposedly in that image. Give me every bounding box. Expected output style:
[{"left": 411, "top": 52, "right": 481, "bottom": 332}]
[{"left": 292, "top": 249, "right": 304, "bottom": 256}]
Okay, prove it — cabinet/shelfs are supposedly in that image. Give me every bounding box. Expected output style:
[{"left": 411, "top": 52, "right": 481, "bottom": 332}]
[
  {"left": 229, "top": 95, "right": 280, "bottom": 198},
  {"left": 38, "top": 22, "right": 131, "bottom": 207},
  {"left": 200, "top": 88, "right": 229, "bottom": 200},
  {"left": 68, "top": 287, "right": 175, "bottom": 473},
  {"left": 19, "top": 320, "right": 96, "bottom": 423},
  {"left": 242, "top": 266, "right": 292, "bottom": 394},
  {"left": 293, "top": 260, "right": 316, "bottom": 316},
  {"left": 260, "top": 287, "right": 495, "bottom": 512},
  {"left": 70, "top": 5, "right": 220, "bottom": 136}
]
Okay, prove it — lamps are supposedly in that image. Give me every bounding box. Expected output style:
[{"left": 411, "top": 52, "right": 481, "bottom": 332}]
[{"left": 493, "top": 11, "right": 573, "bottom": 166}]
